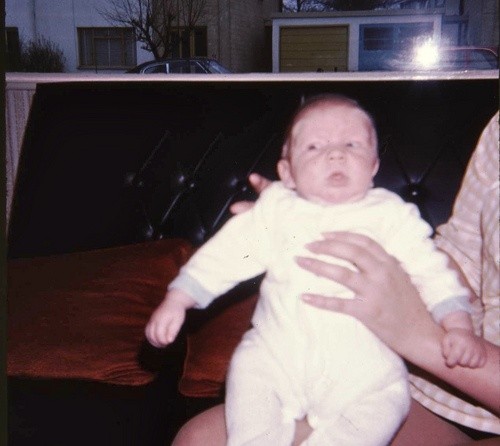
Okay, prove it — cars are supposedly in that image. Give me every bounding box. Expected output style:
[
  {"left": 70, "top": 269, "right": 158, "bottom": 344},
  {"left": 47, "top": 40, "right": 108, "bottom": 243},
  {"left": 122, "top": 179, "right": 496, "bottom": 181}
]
[{"left": 125, "top": 57, "right": 232, "bottom": 74}]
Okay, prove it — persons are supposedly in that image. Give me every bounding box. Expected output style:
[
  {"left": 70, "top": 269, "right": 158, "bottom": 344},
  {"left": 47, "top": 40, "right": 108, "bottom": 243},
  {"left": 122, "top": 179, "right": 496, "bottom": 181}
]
[
  {"left": 172, "top": 97, "right": 498, "bottom": 446},
  {"left": 146, "top": 93, "right": 489, "bottom": 446}
]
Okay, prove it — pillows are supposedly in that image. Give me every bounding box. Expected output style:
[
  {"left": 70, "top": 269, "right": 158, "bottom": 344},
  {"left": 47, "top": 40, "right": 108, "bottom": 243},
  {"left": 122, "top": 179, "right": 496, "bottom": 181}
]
[
  {"left": 176, "top": 285, "right": 257, "bottom": 397},
  {"left": 4, "top": 237, "right": 196, "bottom": 388}
]
